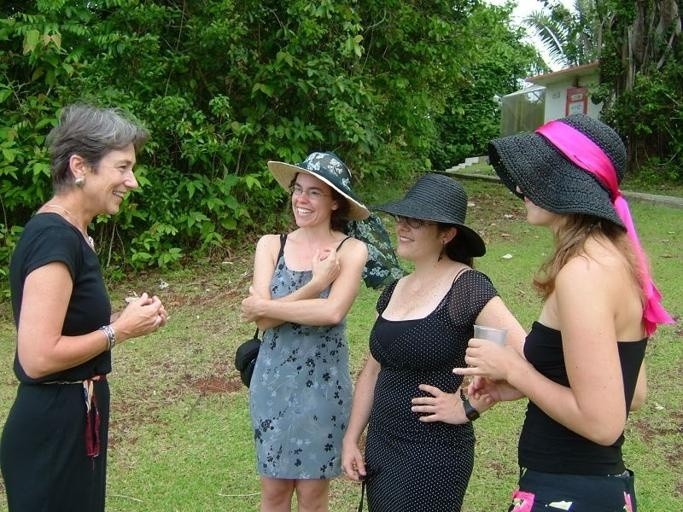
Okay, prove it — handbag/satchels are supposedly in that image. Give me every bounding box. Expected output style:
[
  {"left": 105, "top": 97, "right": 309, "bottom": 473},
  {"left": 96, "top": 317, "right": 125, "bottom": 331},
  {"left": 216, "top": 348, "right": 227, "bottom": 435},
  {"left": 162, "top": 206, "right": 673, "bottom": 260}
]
[{"left": 235, "top": 338, "right": 261, "bottom": 388}]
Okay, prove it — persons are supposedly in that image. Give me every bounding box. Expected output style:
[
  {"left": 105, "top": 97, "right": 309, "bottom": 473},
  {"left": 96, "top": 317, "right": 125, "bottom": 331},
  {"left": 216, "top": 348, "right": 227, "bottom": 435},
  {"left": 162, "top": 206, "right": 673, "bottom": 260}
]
[
  {"left": 240, "top": 147, "right": 372, "bottom": 512},
  {"left": 341, "top": 173, "right": 528, "bottom": 512},
  {"left": 1, "top": 104, "right": 169, "bottom": 512},
  {"left": 451, "top": 115, "right": 675, "bottom": 512}
]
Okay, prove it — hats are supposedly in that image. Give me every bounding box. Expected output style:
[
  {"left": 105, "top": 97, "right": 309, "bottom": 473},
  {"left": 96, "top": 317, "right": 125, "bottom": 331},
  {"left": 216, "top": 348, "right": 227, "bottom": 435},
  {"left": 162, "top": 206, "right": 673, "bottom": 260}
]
[
  {"left": 488, "top": 113, "right": 627, "bottom": 233},
  {"left": 372, "top": 174, "right": 486, "bottom": 257},
  {"left": 266, "top": 151, "right": 371, "bottom": 221}
]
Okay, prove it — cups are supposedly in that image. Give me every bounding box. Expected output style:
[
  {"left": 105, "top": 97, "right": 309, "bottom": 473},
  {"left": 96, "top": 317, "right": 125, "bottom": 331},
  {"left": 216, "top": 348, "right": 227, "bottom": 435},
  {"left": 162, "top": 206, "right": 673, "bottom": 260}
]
[{"left": 471, "top": 324, "right": 508, "bottom": 347}]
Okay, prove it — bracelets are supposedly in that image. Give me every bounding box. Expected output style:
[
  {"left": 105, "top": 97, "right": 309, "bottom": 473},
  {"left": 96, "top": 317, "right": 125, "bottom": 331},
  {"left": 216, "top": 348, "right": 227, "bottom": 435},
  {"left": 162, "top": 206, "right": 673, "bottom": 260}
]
[{"left": 101, "top": 324, "right": 116, "bottom": 352}]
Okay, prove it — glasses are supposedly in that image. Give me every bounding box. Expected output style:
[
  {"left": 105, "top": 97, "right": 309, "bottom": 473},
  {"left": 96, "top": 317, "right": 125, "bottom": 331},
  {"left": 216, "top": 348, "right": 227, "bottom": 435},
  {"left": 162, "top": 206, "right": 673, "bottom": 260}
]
[
  {"left": 394, "top": 214, "right": 439, "bottom": 229},
  {"left": 290, "top": 187, "right": 331, "bottom": 200}
]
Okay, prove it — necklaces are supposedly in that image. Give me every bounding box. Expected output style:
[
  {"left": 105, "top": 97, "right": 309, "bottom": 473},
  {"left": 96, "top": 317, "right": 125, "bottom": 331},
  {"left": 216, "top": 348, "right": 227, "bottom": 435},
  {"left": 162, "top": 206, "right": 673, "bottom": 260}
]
[{"left": 46, "top": 203, "right": 96, "bottom": 248}]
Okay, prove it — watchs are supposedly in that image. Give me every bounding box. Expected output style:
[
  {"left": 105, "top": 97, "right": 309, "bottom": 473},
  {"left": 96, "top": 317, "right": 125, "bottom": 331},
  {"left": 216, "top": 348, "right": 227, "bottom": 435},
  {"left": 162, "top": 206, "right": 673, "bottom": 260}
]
[{"left": 463, "top": 398, "right": 479, "bottom": 422}]
